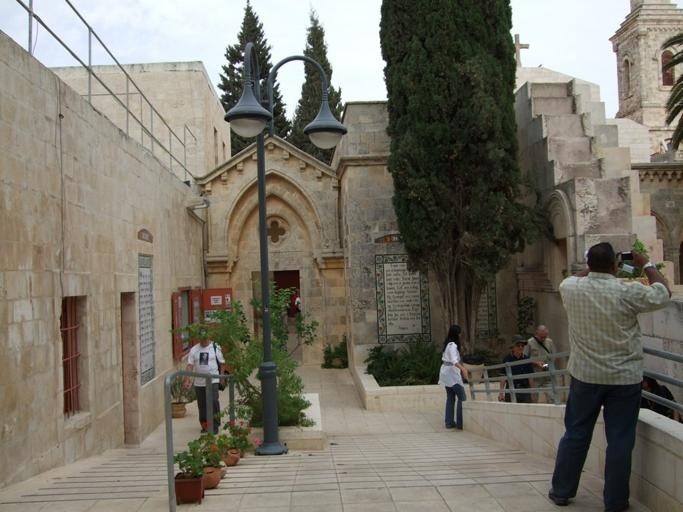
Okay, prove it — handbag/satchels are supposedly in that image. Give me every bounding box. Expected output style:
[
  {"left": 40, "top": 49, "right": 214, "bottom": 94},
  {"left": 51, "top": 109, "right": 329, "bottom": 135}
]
[{"left": 218, "top": 368, "right": 230, "bottom": 390}]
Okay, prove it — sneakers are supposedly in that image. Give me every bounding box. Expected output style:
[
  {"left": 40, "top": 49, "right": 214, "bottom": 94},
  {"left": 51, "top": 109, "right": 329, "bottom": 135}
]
[{"left": 548, "top": 488, "right": 568, "bottom": 506}]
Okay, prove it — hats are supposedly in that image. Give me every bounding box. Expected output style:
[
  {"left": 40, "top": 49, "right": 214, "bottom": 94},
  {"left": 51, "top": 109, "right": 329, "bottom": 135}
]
[{"left": 508, "top": 335, "right": 528, "bottom": 349}]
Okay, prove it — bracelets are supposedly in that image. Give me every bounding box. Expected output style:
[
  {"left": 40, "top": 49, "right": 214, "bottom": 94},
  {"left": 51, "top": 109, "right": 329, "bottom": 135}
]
[{"left": 640, "top": 260, "right": 657, "bottom": 270}]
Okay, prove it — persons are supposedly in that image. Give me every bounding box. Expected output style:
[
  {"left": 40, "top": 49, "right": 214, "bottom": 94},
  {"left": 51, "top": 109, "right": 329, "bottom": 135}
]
[
  {"left": 543, "top": 240, "right": 672, "bottom": 510},
  {"left": 437, "top": 324, "right": 469, "bottom": 431},
  {"left": 520, "top": 325, "right": 565, "bottom": 405},
  {"left": 280, "top": 300, "right": 291, "bottom": 333},
  {"left": 639, "top": 375, "right": 683, "bottom": 424},
  {"left": 180, "top": 328, "right": 227, "bottom": 435},
  {"left": 496, "top": 333, "right": 538, "bottom": 403}
]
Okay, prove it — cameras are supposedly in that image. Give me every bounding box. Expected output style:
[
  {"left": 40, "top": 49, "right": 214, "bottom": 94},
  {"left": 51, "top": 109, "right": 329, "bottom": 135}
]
[
  {"left": 542, "top": 363, "right": 549, "bottom": 369},
  {"left": 621, "top": 251, "right": 633, "bottom": 261}
]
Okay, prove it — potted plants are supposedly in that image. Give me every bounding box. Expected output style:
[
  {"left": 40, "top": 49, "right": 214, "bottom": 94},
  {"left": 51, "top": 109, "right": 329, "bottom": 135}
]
[{"left": 174, "top": 429, "right": 250, "bottom": 503}]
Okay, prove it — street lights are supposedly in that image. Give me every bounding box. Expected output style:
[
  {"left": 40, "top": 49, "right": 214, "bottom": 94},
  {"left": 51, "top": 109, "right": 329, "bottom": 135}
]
[{"left": 220, "top": 40, "right": 350, "bottom": 458}]
